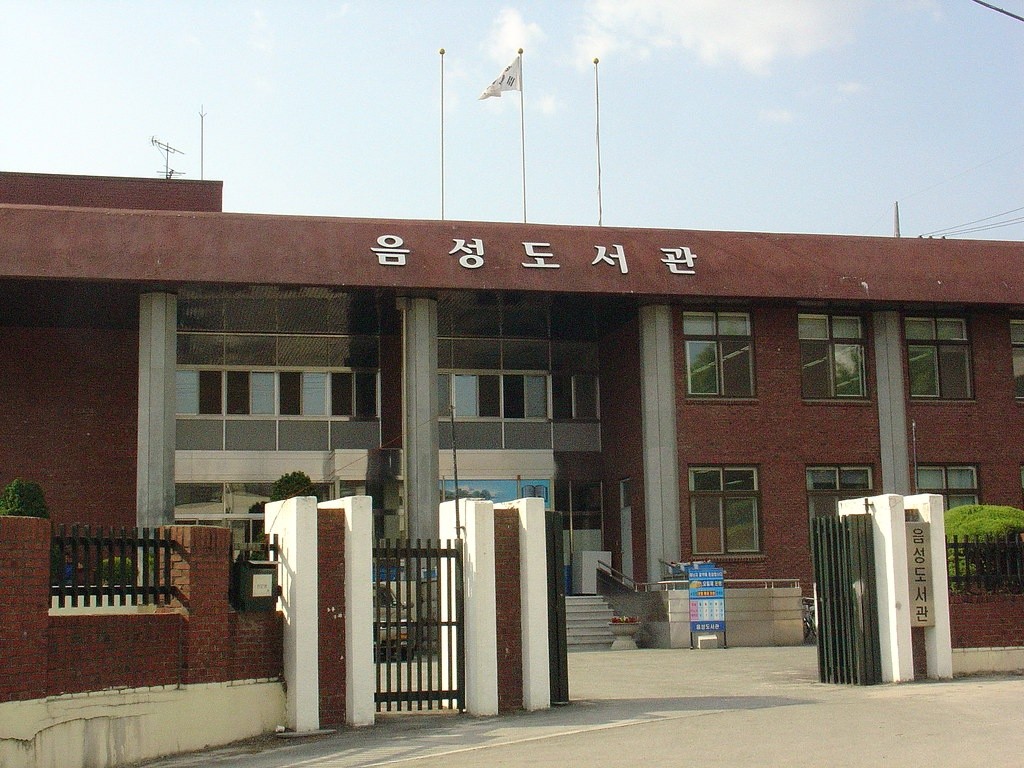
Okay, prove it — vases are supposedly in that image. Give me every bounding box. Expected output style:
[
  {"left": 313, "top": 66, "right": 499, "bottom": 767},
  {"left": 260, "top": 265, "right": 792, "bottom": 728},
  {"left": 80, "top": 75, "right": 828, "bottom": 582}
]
[{"left": 607, "top": 622, "right": 641, "bottom": 649}]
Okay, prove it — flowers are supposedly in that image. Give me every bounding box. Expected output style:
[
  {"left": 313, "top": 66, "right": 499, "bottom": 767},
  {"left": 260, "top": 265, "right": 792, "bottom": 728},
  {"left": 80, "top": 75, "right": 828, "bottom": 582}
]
[{"left": 612, "top": 614, "right": 634, "bottom": 624}]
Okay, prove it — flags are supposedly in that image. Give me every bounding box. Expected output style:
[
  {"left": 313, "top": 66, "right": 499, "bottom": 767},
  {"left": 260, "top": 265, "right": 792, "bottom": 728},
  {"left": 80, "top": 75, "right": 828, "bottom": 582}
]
[{"left": 477, "top": 55, "right": 521, "bottom": 100}]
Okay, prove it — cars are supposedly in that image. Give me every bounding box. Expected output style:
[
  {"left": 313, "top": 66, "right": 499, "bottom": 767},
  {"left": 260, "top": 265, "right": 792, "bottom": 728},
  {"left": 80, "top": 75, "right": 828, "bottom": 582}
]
[{"left": 373, "top": 583, "right": 416, "bottom": 661}]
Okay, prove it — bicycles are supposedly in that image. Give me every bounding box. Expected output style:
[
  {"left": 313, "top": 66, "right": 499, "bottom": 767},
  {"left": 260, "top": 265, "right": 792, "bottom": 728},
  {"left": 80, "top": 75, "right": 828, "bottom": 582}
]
[{"left": 801, "top": 597, "right": 819, "bottom": 645}]
